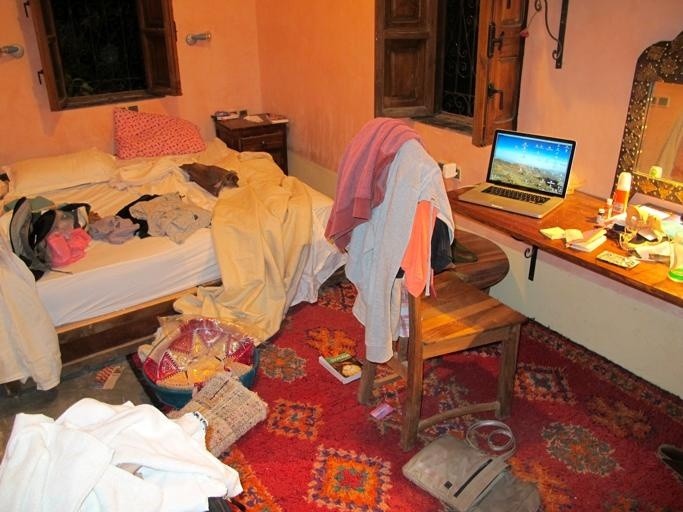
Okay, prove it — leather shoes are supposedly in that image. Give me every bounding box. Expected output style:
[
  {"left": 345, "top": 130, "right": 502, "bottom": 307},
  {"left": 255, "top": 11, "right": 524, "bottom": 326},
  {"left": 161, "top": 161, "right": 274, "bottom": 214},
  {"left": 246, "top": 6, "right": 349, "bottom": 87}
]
[{"left": 451, "top": 238, "right": 478, "bottom": 262}]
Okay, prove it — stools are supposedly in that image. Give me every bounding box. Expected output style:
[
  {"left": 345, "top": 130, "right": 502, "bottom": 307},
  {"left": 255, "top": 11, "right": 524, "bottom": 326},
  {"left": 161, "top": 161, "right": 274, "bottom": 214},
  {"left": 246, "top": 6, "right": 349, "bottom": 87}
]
[{"left": 450, "top": 220, "right": 510, "bottom": 287}]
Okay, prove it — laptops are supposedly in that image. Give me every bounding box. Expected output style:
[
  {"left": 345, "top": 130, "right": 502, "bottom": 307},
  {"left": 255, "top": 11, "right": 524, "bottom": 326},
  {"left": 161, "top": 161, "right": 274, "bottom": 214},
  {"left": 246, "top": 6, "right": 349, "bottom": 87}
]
[{"left": 456, "top": 127, "right": 578, "bottom": 221}]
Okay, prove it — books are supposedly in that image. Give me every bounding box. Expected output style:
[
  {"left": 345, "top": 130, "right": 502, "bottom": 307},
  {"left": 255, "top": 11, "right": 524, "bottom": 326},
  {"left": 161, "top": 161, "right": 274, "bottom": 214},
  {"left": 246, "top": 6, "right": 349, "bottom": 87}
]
[
  {"left": 565, "top": 229, "right": 607, "bottom": 253},
  {"left": 266, "top": 114, "right": 289, "bottom": 125},
  {"left": 319, "top": 352, "right": 362, "bottom": 385}
]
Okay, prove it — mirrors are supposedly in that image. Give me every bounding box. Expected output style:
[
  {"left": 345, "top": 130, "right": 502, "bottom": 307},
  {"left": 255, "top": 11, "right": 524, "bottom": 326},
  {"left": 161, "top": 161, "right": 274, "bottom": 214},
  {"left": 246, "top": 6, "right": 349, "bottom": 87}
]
[{"left": 609, "top": 30, "right": 683, "bottom": 214}]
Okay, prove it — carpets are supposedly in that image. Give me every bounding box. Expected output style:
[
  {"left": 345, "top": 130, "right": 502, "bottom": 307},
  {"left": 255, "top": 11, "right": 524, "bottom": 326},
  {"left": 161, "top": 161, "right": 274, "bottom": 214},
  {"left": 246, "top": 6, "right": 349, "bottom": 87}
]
[{"left": 126, "top": 262, "right": 683, "bottom": 512}]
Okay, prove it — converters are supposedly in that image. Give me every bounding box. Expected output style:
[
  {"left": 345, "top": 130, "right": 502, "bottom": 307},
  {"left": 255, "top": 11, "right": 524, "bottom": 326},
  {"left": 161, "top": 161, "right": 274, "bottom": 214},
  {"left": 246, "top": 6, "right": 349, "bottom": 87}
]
[{"left": 441, "top": 162, "right": 457, "bottom": 179}]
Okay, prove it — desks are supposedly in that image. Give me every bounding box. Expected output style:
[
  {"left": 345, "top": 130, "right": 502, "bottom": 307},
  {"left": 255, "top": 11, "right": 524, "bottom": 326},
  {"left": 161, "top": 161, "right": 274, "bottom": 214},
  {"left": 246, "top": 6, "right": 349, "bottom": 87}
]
[{"left": 440, "top": 181, "right": 683, "bottom": 410}]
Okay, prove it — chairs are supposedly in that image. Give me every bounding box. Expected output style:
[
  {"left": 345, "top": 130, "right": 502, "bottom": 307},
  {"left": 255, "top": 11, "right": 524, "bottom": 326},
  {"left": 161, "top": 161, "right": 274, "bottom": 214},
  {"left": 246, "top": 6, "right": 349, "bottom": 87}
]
[{"left": 357, "top": 130, "right": 528, "bottom": 450}]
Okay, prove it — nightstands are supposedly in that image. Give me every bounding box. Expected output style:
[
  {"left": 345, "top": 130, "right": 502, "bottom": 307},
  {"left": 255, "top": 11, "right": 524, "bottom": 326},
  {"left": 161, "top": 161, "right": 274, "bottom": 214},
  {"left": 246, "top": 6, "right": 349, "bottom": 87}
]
[{"left": 209, "top": 111, "right": 289, "bottom": 180}]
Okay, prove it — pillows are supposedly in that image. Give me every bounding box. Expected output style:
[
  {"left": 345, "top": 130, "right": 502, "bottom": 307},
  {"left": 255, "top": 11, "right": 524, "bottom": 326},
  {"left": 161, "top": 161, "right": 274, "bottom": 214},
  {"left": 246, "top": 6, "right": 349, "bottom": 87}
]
[
  {"left": 111, "top": 102, "right": 207, "bottom": 159},
  {"left": 1, "top": 147, "right": 114, "bottom": 206}
]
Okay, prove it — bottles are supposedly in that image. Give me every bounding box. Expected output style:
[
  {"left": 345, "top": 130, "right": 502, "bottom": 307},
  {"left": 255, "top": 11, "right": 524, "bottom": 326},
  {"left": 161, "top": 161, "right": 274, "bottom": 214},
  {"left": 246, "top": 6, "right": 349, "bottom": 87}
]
[
  {"left": 595, "top": 208, "right": 605, "bottom": 223},
  {"left": 605, "top": 198, "right": 613, "bottom": 218},
  {"left": 668, "top": 214, "right": 683, "bottom": 283},
  {"left": 612, "top": 171, "right": 632, "bottom": 214}
]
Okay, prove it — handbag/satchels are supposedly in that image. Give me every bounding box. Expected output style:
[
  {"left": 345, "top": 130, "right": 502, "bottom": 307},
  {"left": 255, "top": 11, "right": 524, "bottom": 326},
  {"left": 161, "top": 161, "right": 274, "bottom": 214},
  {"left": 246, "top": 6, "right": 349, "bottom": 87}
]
[{"left": 402, "top": 434, "right": 541, "bottom": 511}]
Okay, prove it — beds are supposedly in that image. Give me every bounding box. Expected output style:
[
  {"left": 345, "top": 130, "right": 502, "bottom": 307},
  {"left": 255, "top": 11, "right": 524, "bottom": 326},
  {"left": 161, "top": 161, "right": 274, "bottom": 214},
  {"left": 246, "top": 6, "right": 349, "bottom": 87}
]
[{"left": 0, "top": 140, "right": 342, "bottom": 399}]
